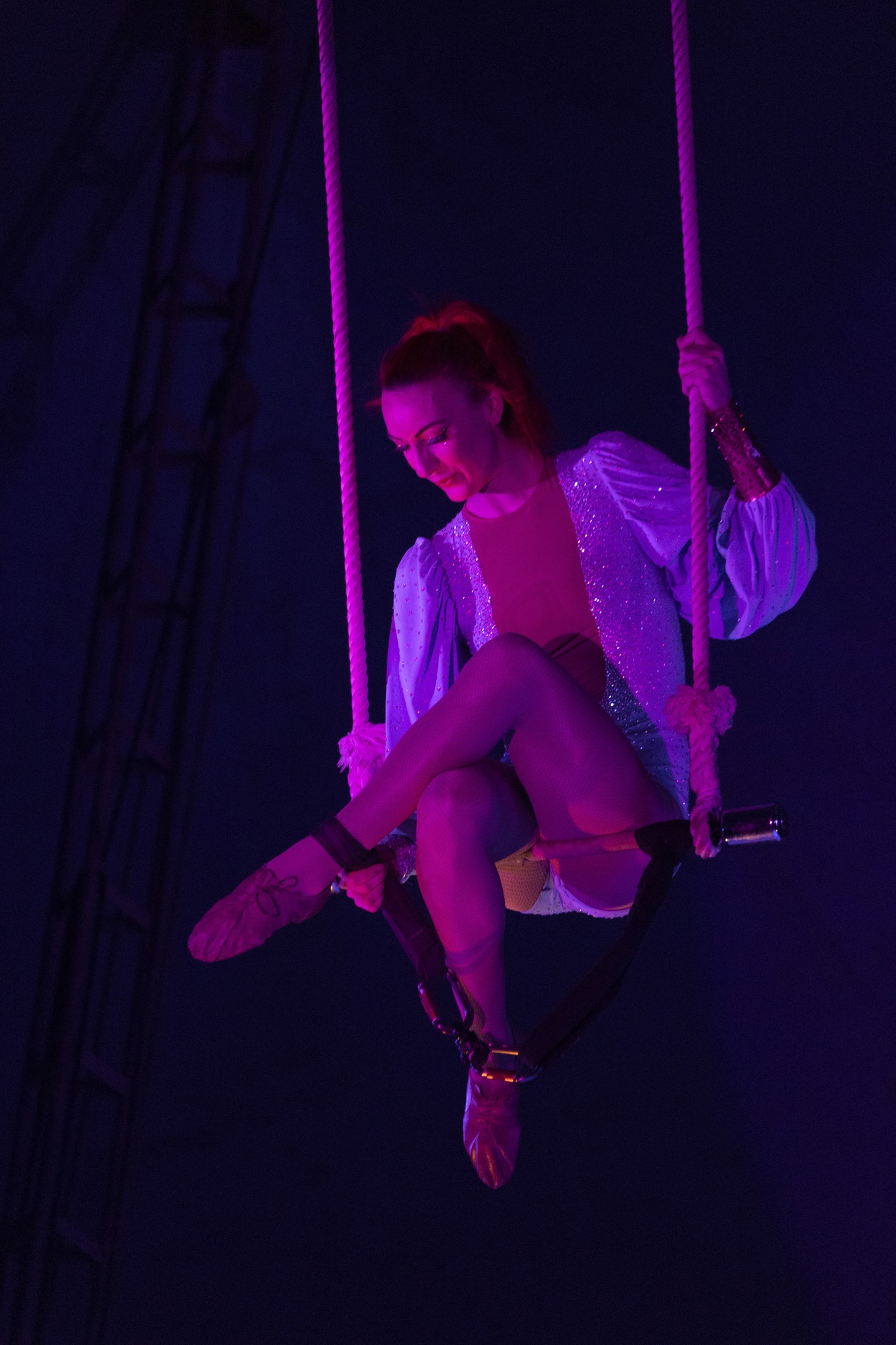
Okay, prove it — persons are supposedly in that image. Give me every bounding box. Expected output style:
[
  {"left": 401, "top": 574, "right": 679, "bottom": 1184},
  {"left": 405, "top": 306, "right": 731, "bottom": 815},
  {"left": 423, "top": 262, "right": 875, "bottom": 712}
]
[{"left": 187, "top": 302, "right": 819, "bottom": 1189}]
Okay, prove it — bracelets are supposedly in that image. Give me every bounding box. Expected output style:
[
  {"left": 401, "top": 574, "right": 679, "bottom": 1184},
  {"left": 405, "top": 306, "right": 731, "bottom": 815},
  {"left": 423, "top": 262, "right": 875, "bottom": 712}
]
[{"left": 386, "top": 836, "right": 414, "bottom": 884}]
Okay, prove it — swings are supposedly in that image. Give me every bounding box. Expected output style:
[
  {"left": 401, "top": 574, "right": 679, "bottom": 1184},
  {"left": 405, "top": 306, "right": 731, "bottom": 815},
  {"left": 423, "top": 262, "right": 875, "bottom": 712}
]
[{"left": 313, "top": 1, "right": 784, "bottom": 881}]
